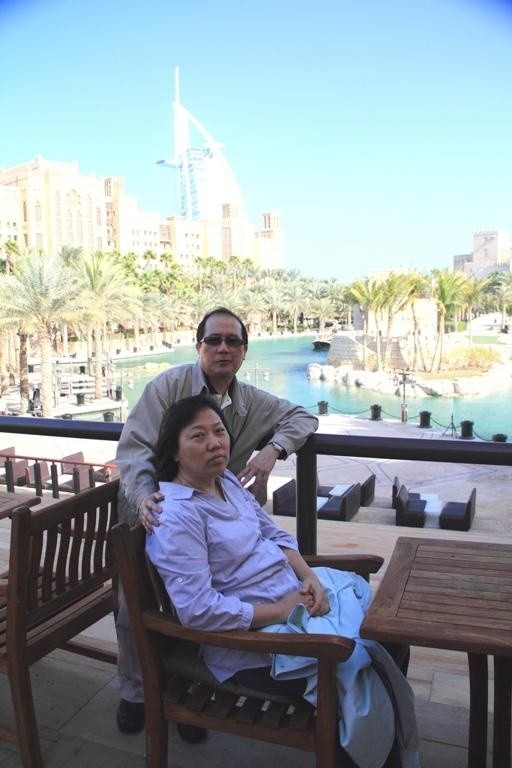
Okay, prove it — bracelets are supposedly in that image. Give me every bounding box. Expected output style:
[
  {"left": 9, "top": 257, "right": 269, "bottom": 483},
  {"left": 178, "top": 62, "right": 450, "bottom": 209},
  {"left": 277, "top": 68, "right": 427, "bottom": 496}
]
[{"left": 271, "top": 441, "right": 285, "bottom": 458}]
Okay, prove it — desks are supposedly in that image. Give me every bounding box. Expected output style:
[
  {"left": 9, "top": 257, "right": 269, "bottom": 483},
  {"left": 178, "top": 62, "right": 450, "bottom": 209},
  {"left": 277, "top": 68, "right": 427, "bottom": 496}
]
[
  {"left": 329, "top": 485, "right": 351, "bottom": 497},
  {"left": 1, "top": 492, "right": 41, "bottom": 520},
  {"left": 421, "top": 493, "right": 438, "bottom": 502},
  {"left": 424, "top": 500, "right": 442, "bottom": 528},
  {"left": 317, "top": 496, "right": 329, "bottom": 510}
]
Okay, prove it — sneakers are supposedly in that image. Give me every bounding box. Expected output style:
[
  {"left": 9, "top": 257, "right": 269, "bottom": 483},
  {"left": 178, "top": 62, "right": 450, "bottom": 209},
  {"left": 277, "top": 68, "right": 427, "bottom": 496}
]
[
  {"left": 116, "top": 696, "right": 147, "bottom": 733},
  {"left": 177, "top": 720, "right": 210, "bottom": 742}
]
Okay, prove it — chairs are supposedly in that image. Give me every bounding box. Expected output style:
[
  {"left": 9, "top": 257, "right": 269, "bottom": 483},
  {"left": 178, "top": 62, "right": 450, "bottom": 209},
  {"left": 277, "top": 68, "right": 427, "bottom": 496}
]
[
  {"left": 361, "top": 474, "right": 375, "bottom": 507},
  {"left": 316, "top": 478, "right": 333, "bottom": 497},
  {"left": 273, "top": 479, "right": 296, "bottom": 516},
  {"left": 1, "top": 446, "right": 116, "bottom": 493},
  {"left": 396, "top": 485, "right": 427, "bottom": 528},
  {"left": 107, "top": 523, "right": 384, "bottom": 768},
  {"left": 392, "top": 476, "right": 420, "bottom": 510},
  {"left": 248, "top": 482, "right": 268, "bottom": 507},
  {"left": 2, "top": 478, "right": 121, "bottom": 768},
  {"left": 438, "top": 488, "right": 476, "bottom": 532},
  {"left": 317, "top": 483, "right": 362, "bottom": 522}
]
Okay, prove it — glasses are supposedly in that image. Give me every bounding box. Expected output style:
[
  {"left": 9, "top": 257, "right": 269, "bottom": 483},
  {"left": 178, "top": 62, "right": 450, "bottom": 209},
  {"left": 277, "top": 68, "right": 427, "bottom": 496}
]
[{"left": 200, "top": 335, "right": 245, "bottom": 346}]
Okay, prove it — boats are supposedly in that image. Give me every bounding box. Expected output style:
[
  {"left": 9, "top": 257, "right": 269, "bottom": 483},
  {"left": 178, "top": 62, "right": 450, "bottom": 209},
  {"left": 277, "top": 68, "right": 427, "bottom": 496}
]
[{"left": 311, "top": 336, "right": 332, "bottom": 350}]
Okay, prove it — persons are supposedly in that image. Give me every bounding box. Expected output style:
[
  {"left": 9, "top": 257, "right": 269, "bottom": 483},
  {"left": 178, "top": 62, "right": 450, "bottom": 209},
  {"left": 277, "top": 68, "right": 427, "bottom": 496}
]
[
  {"left": 142, "top": 394, "right": 419, "bottom": 768},
  {"left": 112, "top": 305, "right": 320, "bottom": 743}
]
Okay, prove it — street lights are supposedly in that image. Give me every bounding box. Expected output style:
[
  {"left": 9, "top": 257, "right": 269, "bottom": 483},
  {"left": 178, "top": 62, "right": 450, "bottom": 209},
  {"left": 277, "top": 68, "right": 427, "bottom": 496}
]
[
  {"left": 398, "top": 366, "right": 412, "bottom": 422},
  {"left": 243, "top": 360, "right": 271, "bottom": 390}
]
